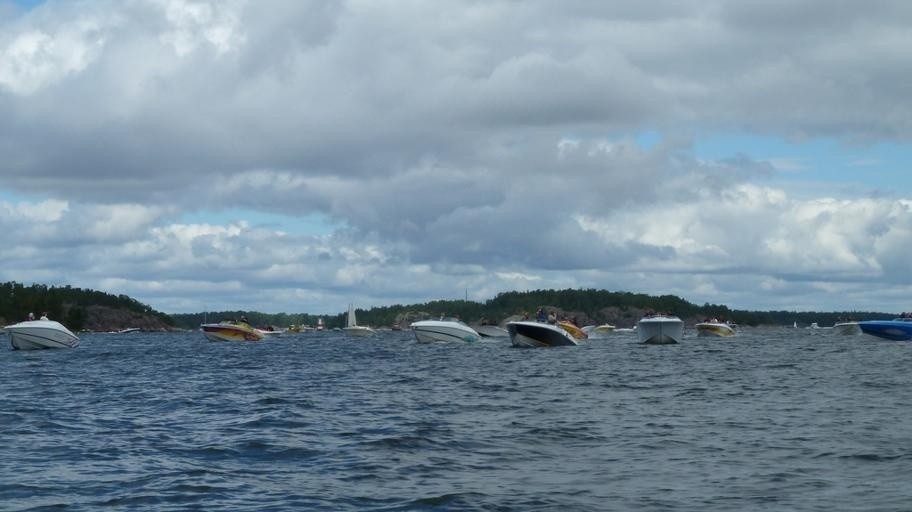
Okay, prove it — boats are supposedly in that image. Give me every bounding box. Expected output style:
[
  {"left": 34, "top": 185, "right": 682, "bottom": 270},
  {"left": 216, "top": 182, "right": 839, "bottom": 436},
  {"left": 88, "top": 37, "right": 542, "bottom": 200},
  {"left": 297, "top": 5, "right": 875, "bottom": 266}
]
[
  {"left": 409, "top": 316, "right": 481, "bottom": 343},
  {"left": 199, "top": 319, "right": 264, "bottom": 343},
  {"left": 110, "top": 325, "right": 141, "bottom": 333},
  {"left": 632, "top": 314, "right": 684, "bottom": 344},
  {"left": 256, "top": 326, "right": 282, "bottom": 337},
  {"left": 833, "top": 321, "right": 860, "bottom": 333},
  {"left": 595, "top": 323, "right": 615, "bottom": 334},
  {"left": 727, "top": 324, "right": 736, "bottom": 329},
  {"left": 3, "top": 310, "right": 79, "bottom": 352},
  {"left": 506, "top": 321, "right": 577, "bottom": 347},
  {"left": 859, "top": 318, "right": 912, "bottom": 341},
  {"left": 558, "top": 321, "right": 588, "bottom": 339},
  {"left": 805, "top": 323, "right": 832, "bottom": 331},
  {"left": 392, "top": 322, "right": 401, "bottom": 330},
  {"left": 580, "top": 325, "right": 595, "bottom": 335},
  {"left": 289, "top": 324, "right": 315, "bottom": 333},
  {"left": 333, "top": 327, "right": 340, "bottom": 332},
  {"left": 695, "top": 323, "right": 736, "bottom": 336}
]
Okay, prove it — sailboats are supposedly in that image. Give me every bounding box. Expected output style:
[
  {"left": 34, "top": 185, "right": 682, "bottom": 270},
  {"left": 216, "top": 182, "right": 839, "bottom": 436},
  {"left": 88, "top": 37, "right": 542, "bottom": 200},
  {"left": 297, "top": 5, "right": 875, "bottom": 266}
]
[
  {"left": 786, "top": 321, "right": 797, "bottom": 329},
  {"left": 316, "top": 318, "right": 324, "bottom": 331},
  {"left": 343, "top": 303, "right": 376, "bottom": 337}
]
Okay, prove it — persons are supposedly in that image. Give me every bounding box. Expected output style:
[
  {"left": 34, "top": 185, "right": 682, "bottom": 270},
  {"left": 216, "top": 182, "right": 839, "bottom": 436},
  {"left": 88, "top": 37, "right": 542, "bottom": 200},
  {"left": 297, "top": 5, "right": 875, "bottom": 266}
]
[
  {"left": 520, "top": 306, "right": 587, "bottom": 327},
  {"left": 645, "top": 308, "right": 675, "bottom": 317},
  {"left": 40, "top": 311, "right": 48, "bottom": 321},
  {"left": 704, "top": 314, "right": 737, "bottom": 325},
  {"left": 27, "top": 312, "right": 35, "bottom": 321},
  {"left": 240, "top": 314, "right": 248, "bottom": 324}
]
